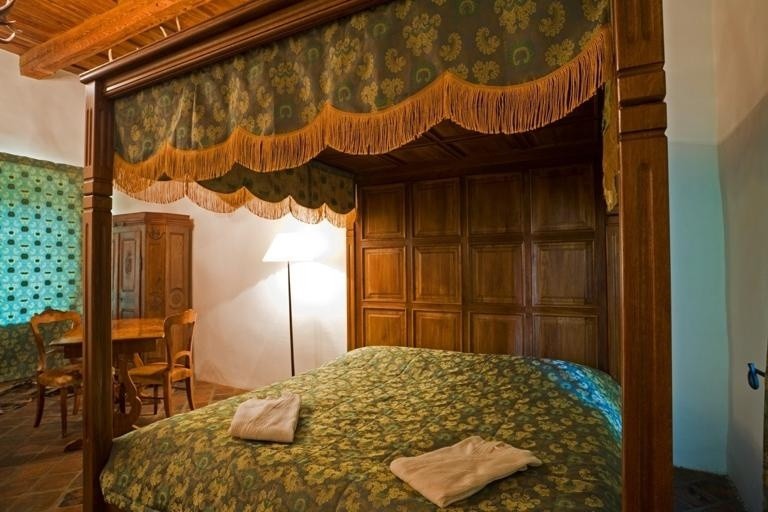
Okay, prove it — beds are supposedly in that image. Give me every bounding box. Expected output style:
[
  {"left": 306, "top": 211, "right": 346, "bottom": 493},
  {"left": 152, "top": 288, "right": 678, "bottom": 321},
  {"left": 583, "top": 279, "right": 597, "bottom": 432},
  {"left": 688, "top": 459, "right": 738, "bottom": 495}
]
[{"left": 79, "top": 0, "right": 674, "bottom": 511}]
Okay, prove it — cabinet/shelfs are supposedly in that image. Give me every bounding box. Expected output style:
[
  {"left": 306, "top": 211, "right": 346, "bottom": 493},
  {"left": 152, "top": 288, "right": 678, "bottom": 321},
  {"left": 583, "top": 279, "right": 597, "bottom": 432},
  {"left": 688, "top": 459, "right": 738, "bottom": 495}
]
[{"left": 110, "top": 211, "right": 195, "bottom": 384}]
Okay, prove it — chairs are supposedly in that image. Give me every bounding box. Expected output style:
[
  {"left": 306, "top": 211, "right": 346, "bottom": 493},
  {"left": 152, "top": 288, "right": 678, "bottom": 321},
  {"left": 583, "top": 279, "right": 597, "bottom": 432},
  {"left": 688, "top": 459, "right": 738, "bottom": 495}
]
[
  {"left": 30, "top": 308, "right": 115, "bottom": 439},
  {"left": 118, "top": 306, "right": 196, "bottom": 418}
]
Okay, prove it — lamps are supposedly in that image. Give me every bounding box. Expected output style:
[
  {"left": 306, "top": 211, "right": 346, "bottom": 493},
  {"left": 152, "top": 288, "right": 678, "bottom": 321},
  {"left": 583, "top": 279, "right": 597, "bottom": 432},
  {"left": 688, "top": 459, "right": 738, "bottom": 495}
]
[{"left": 261, "top": 231, "right": 315, "bottom": 375}]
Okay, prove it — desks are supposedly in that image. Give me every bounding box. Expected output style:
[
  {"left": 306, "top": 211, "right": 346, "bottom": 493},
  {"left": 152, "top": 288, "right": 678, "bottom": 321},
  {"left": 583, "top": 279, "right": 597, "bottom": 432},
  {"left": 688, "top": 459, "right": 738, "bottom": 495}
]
[{"left": 48, "top": 316, "right": 169, "bottom": 452}]
